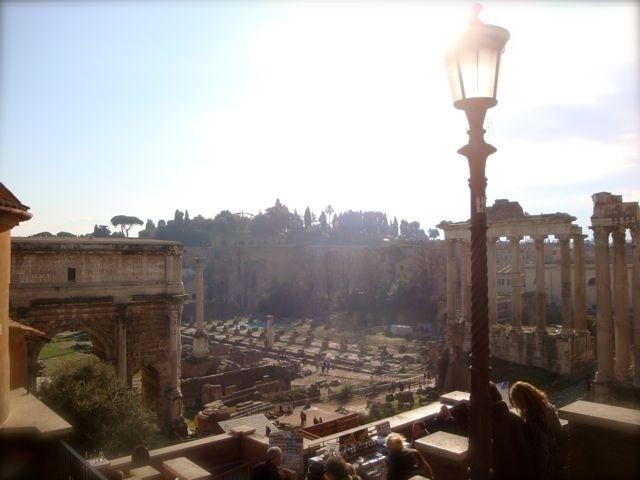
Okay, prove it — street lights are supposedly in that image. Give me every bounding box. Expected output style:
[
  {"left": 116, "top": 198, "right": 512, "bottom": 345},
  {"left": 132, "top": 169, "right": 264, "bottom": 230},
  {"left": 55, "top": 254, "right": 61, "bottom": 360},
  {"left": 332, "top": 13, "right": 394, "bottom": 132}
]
[{"left": 436, "top": 0, "right": 516, "bottom": 480}]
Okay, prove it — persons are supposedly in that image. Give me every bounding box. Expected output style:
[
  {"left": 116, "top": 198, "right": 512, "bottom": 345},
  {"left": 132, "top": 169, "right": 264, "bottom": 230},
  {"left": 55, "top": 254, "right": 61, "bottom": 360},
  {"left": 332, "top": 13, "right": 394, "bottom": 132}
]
[
  {"left": 250, "top": 446, "right": 283, "bottom": 480},
  {"left": 300, "top": 411, "right": 307, "bottom": 424},
  {"left": 383, "top": 431, "right": 433, "bottom": 480},
  {"left": 110, "top": 469, "right": 125, "bottom": 480},
  {"left": 304, "top": 454, "right": 364, "bottom": 480},
  {"left": 279, "top": 403, "right": 294, "bottom": 416},
  {"left": 264, "top": 425, "right": 271, "bottom": 437},
  {"left": 131, "top": 439, "right": 151, "bottom": 465},
  {"left": 387, "top": 379, "right": 411, "bottom": 393},
  {"left": 509, "top": 381, "right": 565, "bottom": 480},
  {"left": 470, "top": 381, "right": 533, "bottom": 480},
  {"left": 319, "top": 417, "right": 322, "bottom": 423},
  {"left": 431, "top": 406, "right": 458, "bottom": 434},
  {"left": 313, "top": 416, "right": 318, "bottom": 424},
  {"left": 410, "top": 420, "right": 430, "bottom": 444}
]
